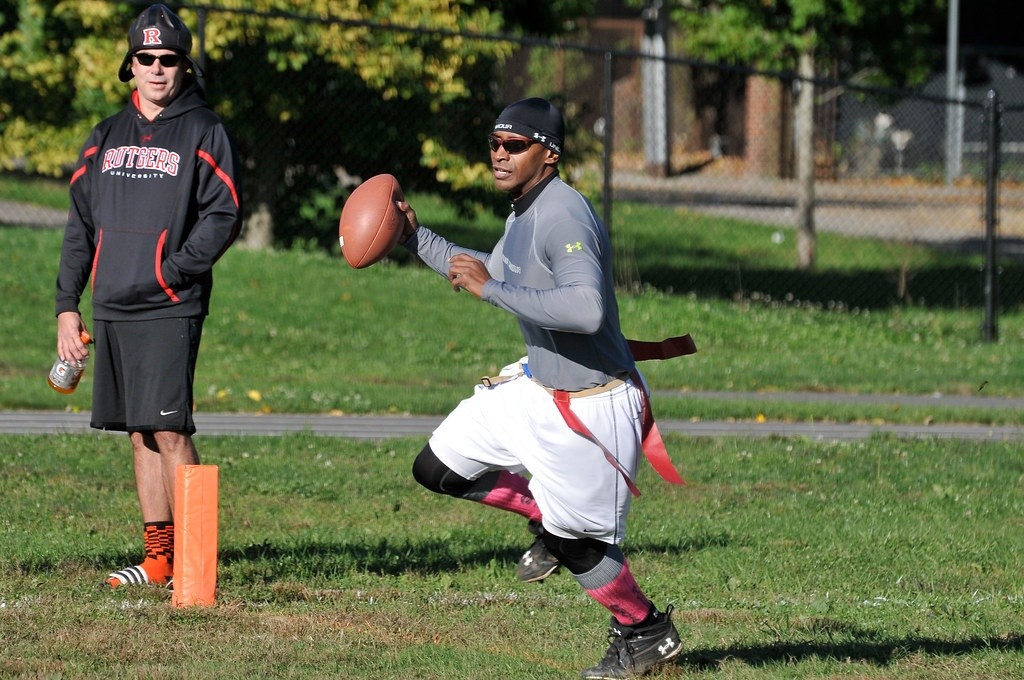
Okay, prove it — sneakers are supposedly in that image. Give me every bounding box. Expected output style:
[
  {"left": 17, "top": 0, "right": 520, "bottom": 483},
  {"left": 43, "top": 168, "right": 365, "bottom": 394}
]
[
  {"left": 517, "top": 519, "right": 562, "bottom": 584},
  {"left": 583, "top": 604, "right": 683, "bottom": 680}
]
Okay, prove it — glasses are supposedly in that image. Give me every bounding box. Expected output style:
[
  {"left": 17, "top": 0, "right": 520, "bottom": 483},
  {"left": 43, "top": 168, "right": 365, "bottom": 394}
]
[
  {"left": 488, "top": 135, "right": 538, "bottom": 155},
  {"left": 132, "top": 53, "right": 179, "bottom": 67}
]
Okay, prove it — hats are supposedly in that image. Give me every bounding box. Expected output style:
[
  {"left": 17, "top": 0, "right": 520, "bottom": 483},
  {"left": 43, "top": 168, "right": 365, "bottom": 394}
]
[
  {"left": 493, "top": 97, "right": 565, "bottom": 156},
  {"left": 118, "top": 3, "right": 203, "bottom": 82}
]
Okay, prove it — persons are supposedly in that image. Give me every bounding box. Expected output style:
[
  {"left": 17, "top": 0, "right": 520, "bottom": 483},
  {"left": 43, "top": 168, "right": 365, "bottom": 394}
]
[
  {"left": 55, "top": 4, "right": 241, "bottom": 588},
  {"left": 399, "top": 98, "right": 696, "bottom": 680}
]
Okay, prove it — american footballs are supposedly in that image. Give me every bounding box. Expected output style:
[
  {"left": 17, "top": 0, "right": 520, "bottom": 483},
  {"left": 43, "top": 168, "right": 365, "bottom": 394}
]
[{"left": 339, "top": 174, "right": 406, "bottom": 270}]
